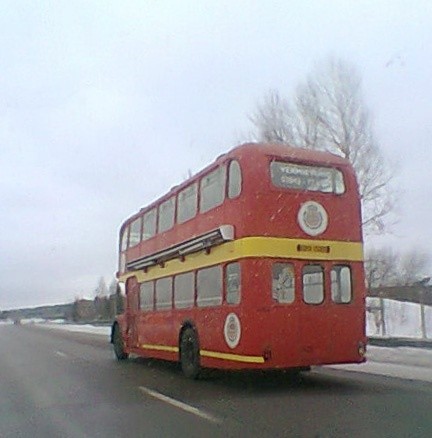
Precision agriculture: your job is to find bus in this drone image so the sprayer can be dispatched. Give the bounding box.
[111,143,367,380]
